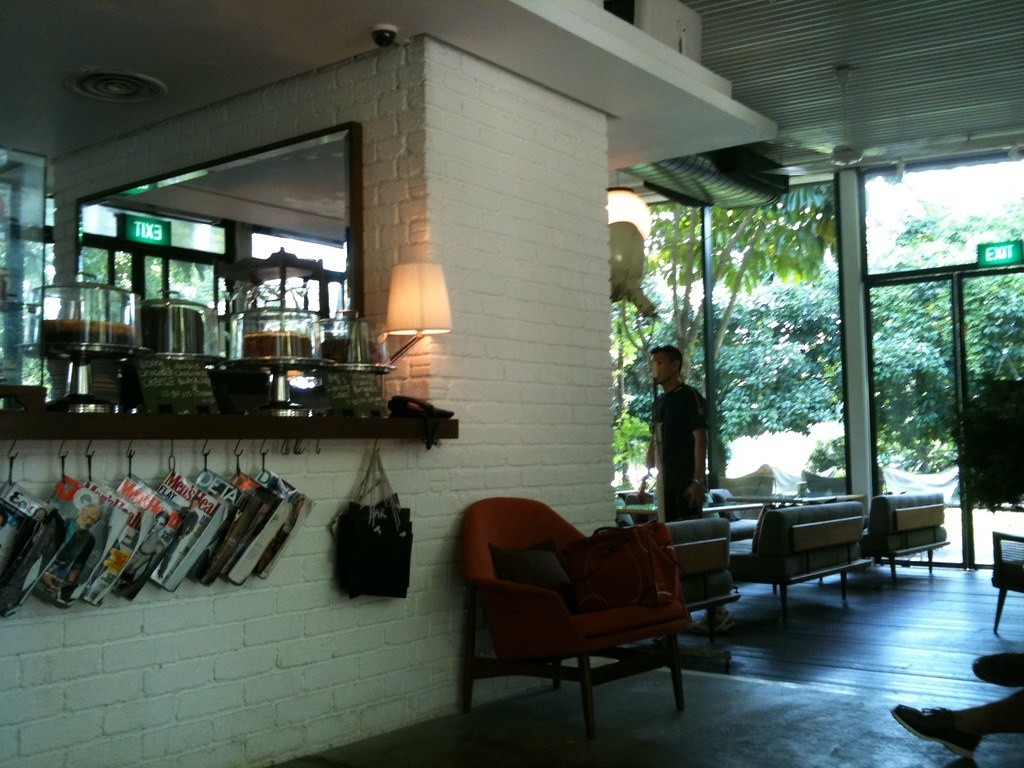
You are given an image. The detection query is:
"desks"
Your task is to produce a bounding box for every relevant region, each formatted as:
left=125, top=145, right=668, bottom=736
left=725, top=493, right=865, bottom=503
left=615, top=503, right=658, bottom=515
left=700, top=503, right=763, bottom=513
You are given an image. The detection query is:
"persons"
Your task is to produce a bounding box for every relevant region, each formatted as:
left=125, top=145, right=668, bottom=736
left=14, top=508, right=46, bottom=570
left=114, top=511, right=169, bottom=597
left=890, top=651, right=1024, bottom=755
left=643, top=345, right=737, bottom=630
left=39, top=502, right=99, bottom=601
left=157, top=497, right=198, bottom=582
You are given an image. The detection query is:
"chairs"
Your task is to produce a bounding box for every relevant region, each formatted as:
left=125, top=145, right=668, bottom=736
left=456, top=495, right=693, bottom=742
left=990, top=530, right=1024, bottom=634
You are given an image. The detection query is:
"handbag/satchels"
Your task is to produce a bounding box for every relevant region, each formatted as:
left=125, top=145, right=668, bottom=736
left=329, top=449, right=413, bottom=598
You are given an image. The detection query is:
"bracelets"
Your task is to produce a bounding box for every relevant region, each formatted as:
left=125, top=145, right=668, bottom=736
left=693, top=478, right=704, bottom=487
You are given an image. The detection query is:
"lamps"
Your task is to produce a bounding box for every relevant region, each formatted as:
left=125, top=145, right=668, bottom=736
left=605, top=168, right=652, bottom=241
left=385, top=261, right=452, bottom=364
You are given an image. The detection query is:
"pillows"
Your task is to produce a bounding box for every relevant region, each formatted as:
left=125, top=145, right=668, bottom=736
left=487, top=535, right=575, bottom=614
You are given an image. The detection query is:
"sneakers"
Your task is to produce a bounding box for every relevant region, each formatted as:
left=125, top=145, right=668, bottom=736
left=890, top=704, right=984, bottom=758
left=973, top=652, right=1024, bottom=686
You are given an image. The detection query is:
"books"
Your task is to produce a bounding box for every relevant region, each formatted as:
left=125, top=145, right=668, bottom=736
left=0, top=469, right=313, bottom=619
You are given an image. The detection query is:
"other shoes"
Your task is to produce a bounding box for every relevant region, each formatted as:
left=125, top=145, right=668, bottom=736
left=688, top=610, right=736, bottom=635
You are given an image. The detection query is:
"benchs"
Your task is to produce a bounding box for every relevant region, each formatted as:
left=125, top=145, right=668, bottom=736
left=615, top=489, right=951, bottom=646
